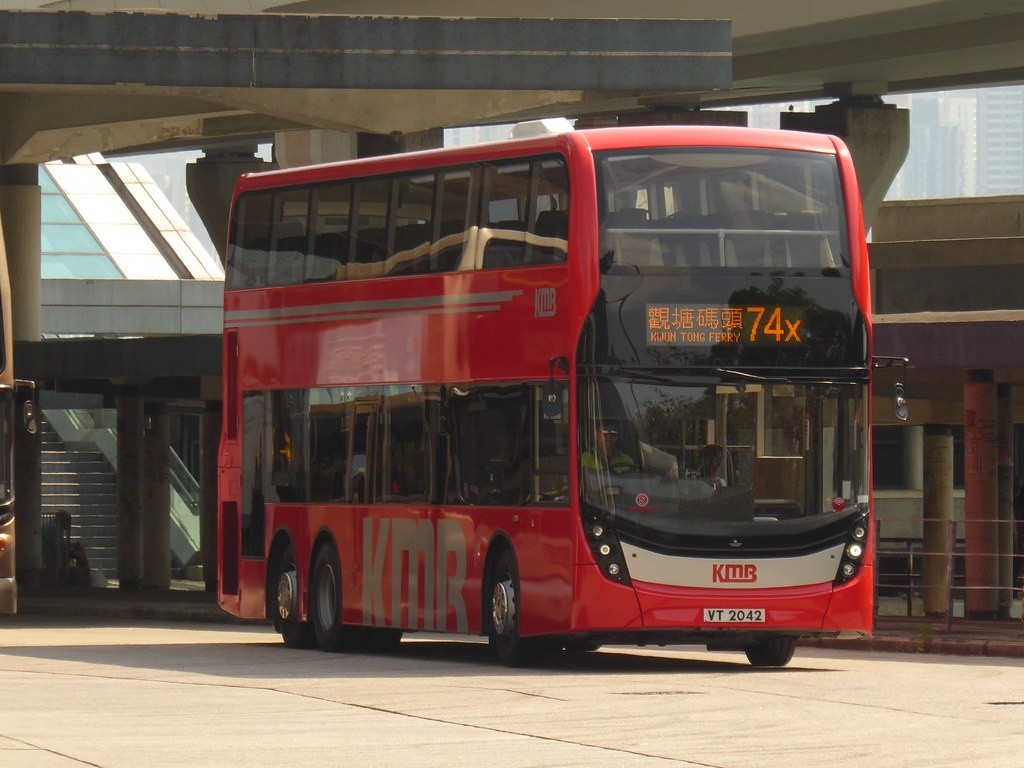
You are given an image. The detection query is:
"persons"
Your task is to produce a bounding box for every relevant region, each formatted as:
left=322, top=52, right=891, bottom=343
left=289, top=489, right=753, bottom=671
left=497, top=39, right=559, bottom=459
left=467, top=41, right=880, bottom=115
left=694, top=445, right=733, bottom=491
left=566, top=422, right=639, bottom=509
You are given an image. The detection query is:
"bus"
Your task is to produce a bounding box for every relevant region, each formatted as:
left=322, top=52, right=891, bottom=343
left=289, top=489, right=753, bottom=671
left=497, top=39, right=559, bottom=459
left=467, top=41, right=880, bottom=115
left=216, top=125, right=910, bottom=668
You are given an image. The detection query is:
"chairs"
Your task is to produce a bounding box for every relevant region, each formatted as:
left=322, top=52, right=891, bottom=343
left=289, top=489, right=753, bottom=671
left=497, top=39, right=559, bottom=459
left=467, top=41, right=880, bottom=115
left=235, top=207, right=821, bottom=289
